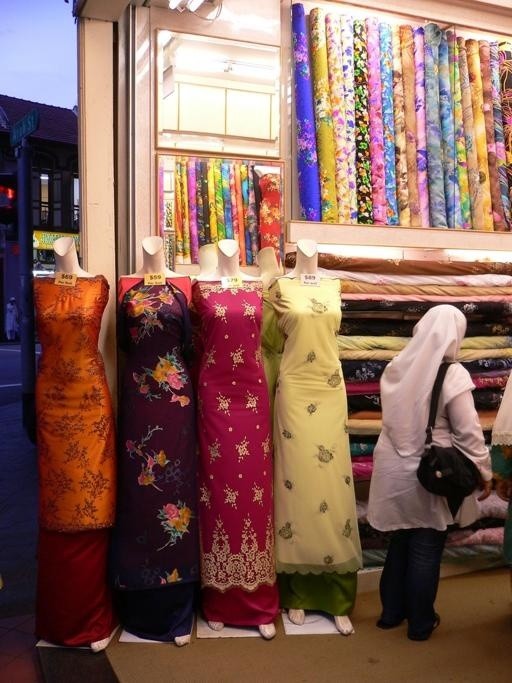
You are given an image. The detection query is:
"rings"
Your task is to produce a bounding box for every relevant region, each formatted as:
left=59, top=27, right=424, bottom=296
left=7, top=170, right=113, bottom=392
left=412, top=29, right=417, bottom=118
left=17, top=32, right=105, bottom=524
left=486, top=486, right=491, bottom=489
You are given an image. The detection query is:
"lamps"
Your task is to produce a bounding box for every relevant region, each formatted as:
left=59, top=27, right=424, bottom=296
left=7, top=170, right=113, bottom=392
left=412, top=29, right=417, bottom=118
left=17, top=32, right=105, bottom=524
left=166, top=0, right=205, bottom=15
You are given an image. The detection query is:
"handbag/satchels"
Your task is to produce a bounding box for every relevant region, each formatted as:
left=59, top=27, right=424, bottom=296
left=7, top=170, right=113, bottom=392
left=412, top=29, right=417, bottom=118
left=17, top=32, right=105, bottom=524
left=415, top=446, right=480, bottom=499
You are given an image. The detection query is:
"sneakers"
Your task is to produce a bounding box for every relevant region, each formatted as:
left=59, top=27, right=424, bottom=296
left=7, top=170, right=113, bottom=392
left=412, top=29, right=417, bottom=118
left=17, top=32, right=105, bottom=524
left=406, top=610, right=441, bottom=642
left=376, top=610, right=405, bottom=630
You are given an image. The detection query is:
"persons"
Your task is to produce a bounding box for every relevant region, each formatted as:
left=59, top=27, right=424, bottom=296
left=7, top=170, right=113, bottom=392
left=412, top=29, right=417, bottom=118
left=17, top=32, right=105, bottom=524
left=491, top=369, right=512, bottom=566
left=4, top=296, right=17, bottom=340
left=252, top=165, right=281, bottom=268
left=269, top=239, right=364, bottom=633
left=189, top=238, right=280, bottom=640
left=115, top=235, right=196, bottom=646
left=367, top=303, right=494, bottom=639
left=30, top=236, right=116, bottom=653
